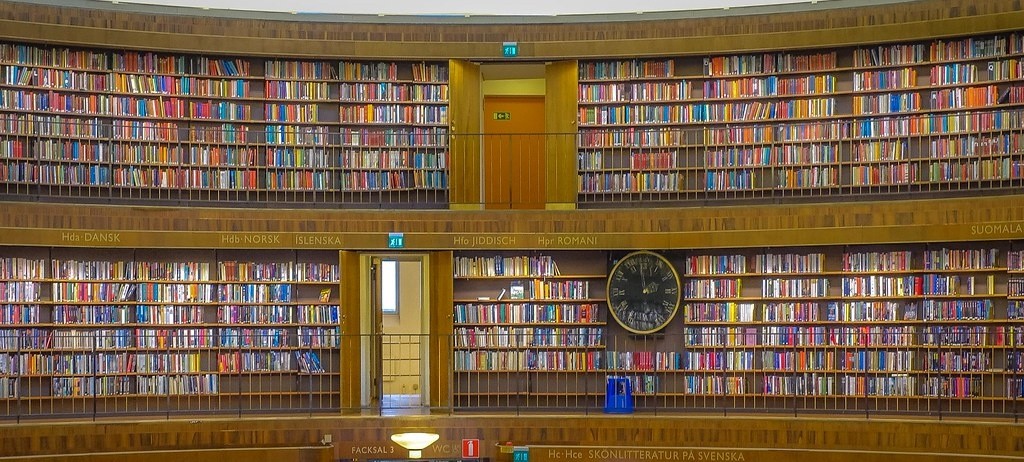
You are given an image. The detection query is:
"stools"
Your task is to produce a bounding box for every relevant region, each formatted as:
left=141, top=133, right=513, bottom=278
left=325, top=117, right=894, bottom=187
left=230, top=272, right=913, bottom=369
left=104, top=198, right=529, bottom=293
left=604, top=377, right=634, bottom=414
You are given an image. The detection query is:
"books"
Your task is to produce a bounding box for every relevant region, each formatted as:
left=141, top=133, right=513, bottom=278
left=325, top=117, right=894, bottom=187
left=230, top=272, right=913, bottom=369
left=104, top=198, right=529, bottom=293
left=218, top=251, right=342, bottom=375
left=1, top=41, right=258, bottom=190
left=607, top=352, right=683, bottom=394
left=0, top=252, right=217, bottom=397
left=454, top=254, right=604, bottom=373
left=266, top=60, right=449, bottom=192
left=695, top=52, right=850, bottom=189
left=578, top=59, right=693, bottom=194
left=853, top=35, right=1024, bottom=185
left=684, top=253, right=1024, bottom=397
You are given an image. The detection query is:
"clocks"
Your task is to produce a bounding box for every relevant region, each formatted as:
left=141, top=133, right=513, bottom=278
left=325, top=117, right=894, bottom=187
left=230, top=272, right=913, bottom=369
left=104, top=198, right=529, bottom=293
left=606, top=251, right=682, bottom=335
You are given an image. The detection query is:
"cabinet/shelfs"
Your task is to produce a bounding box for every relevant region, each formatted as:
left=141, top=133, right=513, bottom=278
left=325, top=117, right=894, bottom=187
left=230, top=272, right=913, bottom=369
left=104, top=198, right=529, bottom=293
left=0, top=29, right=1024, bottom=419
